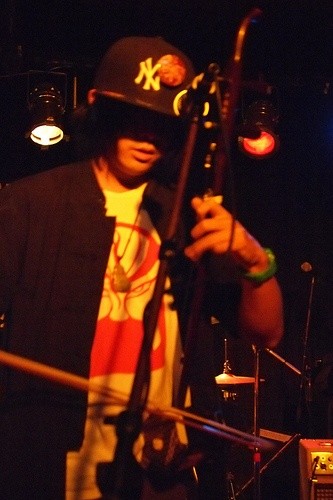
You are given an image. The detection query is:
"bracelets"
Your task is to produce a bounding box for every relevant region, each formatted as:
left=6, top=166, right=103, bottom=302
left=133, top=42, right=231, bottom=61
left=238, top=249, right=278, bottom=283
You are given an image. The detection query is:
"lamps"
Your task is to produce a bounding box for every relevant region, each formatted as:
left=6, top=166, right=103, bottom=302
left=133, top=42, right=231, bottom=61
left=23, top=69, right=71, bottom=153
left=237, top=99, right=283, bottom=161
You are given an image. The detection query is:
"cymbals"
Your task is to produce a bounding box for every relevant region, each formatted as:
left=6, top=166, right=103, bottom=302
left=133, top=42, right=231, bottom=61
left=214, top=373, right=266, bottom=385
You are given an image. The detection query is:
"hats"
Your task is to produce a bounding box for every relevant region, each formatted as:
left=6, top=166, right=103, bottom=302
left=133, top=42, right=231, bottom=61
left=89, top=36, right=196, bottom=123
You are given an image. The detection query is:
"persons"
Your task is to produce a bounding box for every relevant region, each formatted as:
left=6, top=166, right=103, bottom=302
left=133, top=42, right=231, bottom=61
left=0, top=35, right=284, bottom=500
